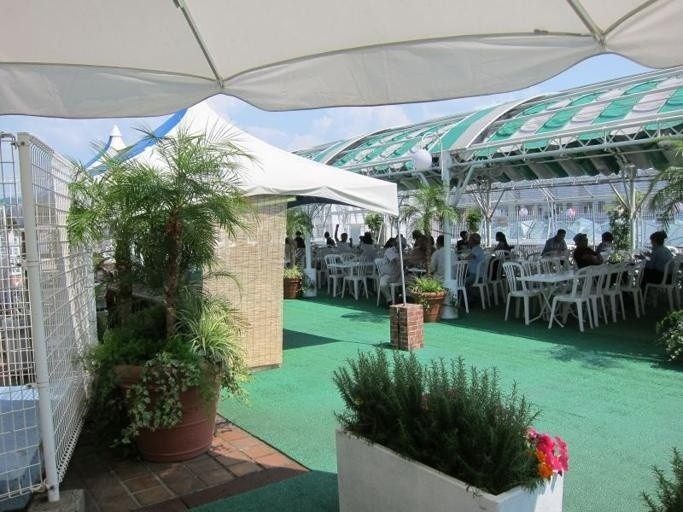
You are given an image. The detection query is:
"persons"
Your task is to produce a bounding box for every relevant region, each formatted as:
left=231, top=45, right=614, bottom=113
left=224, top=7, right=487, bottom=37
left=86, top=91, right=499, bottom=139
left=540, top=228, right=567, bottom=256
left=457, top=231, right=472, bottom=261
left=460, top=232, right=485, bottom=312
left=286, top=223, right=437, bottom=305
left=595, top=231, right=613, bottom=254
left=494, top=231, right=509, bottom=255
left=573, top=232, right=602, bottom=269
left=641, top=230, right=673, bottom=296
left=430, top=235, right=457, bottom=281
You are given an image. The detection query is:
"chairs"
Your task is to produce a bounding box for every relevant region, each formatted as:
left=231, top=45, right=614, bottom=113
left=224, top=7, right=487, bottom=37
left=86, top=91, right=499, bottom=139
left=315, top=246, right=683, bottom=332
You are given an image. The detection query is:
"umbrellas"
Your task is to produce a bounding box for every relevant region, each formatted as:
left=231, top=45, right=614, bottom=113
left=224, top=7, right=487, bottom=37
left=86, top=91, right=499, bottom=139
left=0, top=1, right=683, bottom=121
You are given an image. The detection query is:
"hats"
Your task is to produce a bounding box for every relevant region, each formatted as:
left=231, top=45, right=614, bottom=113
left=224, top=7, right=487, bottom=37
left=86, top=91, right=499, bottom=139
left=470, top=233, right=480, bottom=241
left=396, top=237, right=406, bottom=248
left=573, top=234, right=587, bottom=245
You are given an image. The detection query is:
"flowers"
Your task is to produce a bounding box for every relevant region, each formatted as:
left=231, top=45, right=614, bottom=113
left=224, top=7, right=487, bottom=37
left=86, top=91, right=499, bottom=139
left=332, top=341, right=570, bottom=495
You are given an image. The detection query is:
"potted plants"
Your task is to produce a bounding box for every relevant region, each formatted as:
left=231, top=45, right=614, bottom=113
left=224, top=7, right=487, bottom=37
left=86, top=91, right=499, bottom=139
left=397, top=178, right=464, bottom=323
left=283, top=207, right=314, bottom=299
left=106, top=117, right=264, bottom=463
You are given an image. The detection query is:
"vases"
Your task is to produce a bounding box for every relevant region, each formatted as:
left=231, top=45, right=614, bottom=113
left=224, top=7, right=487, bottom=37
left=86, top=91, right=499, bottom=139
left=335, top=426, right=565, bottom=512
